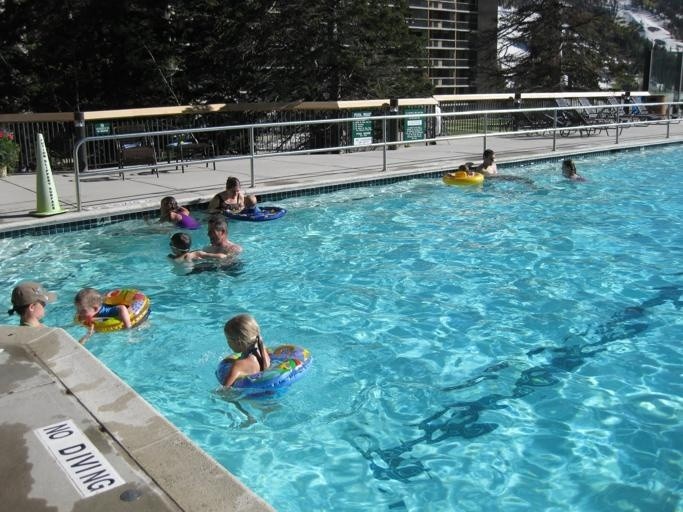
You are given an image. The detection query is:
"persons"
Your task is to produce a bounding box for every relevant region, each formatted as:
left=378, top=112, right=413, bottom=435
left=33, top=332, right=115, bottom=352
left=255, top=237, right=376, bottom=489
left=448, top=164, right=468, bottom=177
left=167, top=233, right=225, bottom=277
left=220, top=314, right=279, bottom=428
left=74, top=287, right=150, bottom=345
left=476, top=149, right=512, bottom=195
left=206, top=177, right=244, bottom=215
left=144, top=195, right=200, bottom=230
left=240, top=195, right=260, bottom=214
left=184, top=218, right=245, bottom=279
left=7, top=281, right=94, bottom=345
left=559, top=159, right=584, bottom=182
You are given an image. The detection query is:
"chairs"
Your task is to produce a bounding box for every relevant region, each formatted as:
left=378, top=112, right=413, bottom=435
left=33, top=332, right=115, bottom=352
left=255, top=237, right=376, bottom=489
left=175, top=115, right=216, bottom=172
left=521, top=95, right=680, bottom=138
left=112, top=124, right=160, bottom=180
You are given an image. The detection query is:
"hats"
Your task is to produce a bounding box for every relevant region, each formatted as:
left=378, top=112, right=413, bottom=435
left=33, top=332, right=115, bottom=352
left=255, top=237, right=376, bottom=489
left=12, top=281, right=57, bottom=309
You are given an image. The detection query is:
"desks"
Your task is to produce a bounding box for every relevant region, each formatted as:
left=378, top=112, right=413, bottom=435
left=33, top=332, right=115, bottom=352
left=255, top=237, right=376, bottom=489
left=165, top=141, right=192, bottom=164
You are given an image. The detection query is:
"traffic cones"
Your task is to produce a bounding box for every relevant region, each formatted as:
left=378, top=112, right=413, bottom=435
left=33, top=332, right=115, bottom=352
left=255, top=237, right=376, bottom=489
left=30, top=132, right=68, bottom=218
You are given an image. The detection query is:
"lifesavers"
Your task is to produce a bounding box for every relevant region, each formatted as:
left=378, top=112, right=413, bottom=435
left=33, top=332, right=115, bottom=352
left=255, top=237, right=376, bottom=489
left=442, top=169, right=484, bottom=185
left=215, top=344, right=314, bottom=391
left=76, top=287, right=150, bottom=332
left=224, top=205, right=286, bottom=221
